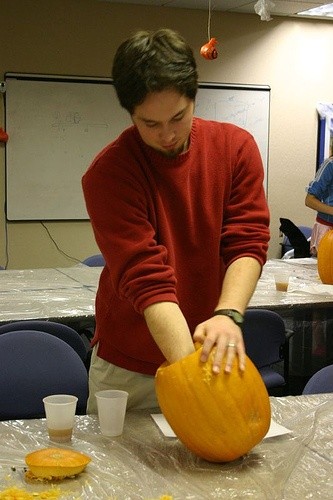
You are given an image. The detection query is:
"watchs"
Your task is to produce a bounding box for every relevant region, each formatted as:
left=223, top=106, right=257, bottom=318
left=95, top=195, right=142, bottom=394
left=214, top=309, right=244, bottom=327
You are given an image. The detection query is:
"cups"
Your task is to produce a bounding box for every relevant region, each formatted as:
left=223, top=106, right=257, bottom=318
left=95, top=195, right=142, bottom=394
left=94, top=390, right=129, bottom=437
left=43, top=394, right=78, bottom=444
left=273, top=271, right=290, bottom=292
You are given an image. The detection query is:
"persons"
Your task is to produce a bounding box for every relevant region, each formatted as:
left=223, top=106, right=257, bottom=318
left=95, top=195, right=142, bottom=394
left=81, top=28, right=270, bottom=415
left=305, top=137, right=333, bottom=258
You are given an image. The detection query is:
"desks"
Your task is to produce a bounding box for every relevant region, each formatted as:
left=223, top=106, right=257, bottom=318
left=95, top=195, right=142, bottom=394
left=0, top=255, right=333, bottom=396
left=0, top=392, right=333, bottom=500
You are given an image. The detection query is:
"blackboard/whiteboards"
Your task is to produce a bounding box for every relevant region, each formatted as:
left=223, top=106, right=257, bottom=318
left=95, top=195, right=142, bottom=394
left=4, top=72, right=269, bottom=222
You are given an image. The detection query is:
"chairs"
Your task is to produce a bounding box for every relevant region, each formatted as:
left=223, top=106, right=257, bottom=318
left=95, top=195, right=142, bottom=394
left=0, top=225, right=333, bottom=421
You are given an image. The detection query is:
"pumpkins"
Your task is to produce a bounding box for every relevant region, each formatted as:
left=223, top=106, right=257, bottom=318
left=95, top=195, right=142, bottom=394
left=317, top=229, right=333, bottom=285
left=155, top=342, right=271, bottom=462
left=25, top=447, right=90, bottom=480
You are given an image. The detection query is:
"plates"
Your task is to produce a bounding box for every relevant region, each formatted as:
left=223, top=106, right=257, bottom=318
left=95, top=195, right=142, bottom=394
left=24, top=447, right=91, bottom=480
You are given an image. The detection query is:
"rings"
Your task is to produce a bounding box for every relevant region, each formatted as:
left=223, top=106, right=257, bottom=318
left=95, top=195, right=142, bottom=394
left=228, top=343, right=237, bottom=348
